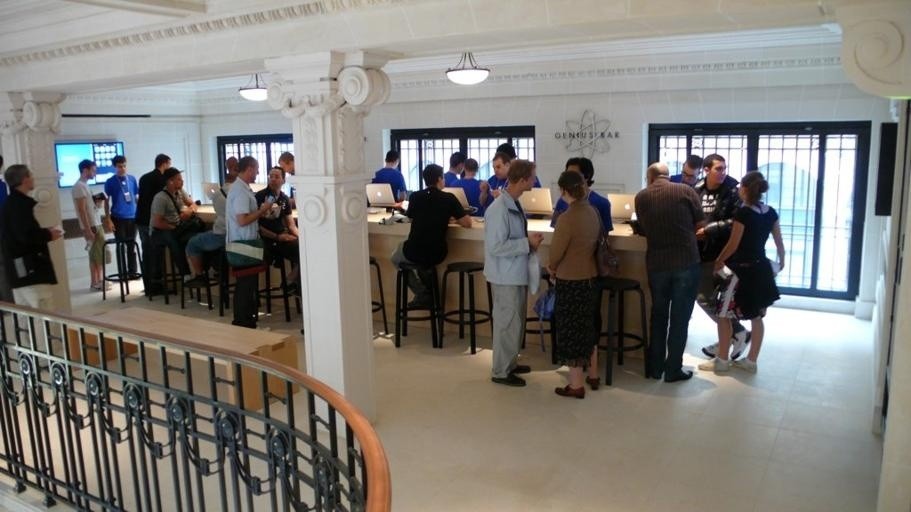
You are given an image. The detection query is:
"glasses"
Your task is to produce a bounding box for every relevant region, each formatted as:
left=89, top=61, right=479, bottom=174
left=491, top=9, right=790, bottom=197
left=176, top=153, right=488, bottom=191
left=681, top=172, right=700, bottom=179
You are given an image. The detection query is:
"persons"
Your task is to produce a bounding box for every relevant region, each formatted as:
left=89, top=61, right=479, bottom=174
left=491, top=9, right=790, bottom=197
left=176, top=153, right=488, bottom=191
left=696, top=154, right=751, bottom=360
left=483, top=159, right=544, bottom=386
left=135, top=154, right=172, bottom=297
left=390, top=164, right=472, bottom=307
left=443, top=144, right=544, bottom=220
left=0, top=164, right=62, bottom=289
left=549, top=170, right=604, bottom=398
left=375, top=150, right=407, bottom=202
left=71, top=159, right=112, bottom=290
left=104, top=156, right=140, bottom=279
left=635, top=162, right=704, bottom=384
left=226, top=156, right=271, bottom=329
left=254, top=166, right=300, bottom=293
left=550, top=157, right=613, bottom=276
left=670, top=156, right=704, bottom=186
left=185, top=167, right=238, bottom=286
left=698, top=171, right=785, bottom=375
left=278, top=152, right=297, bottom=208
left=149, top=166, right=198, bottom=274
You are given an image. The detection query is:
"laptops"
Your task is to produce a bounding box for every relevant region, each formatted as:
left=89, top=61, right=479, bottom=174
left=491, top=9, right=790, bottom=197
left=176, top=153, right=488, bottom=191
left=518, top=188, right=554, bottom=215
left=607, top=192, right=642, bottom=224
left=441, top=187, right=472, bottom=213
left=250, top=184, right=267, bottom=194
left=366, top=183, right=400, bottom=207
left=203, top=182, right=222, bottom=201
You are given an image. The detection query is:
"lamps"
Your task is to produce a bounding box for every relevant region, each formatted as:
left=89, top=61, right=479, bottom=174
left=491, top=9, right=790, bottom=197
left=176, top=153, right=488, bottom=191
left=445, top=51, right=490, bottom=85
left=238, top=73, right=268, bottom=102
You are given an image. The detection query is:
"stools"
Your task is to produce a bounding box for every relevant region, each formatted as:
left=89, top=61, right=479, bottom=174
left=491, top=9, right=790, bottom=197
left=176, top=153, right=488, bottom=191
left=521, top=266, right=556, bottom=365
left=102, top=237, right=389, bottom=335
left=597, top=276, right=651, bottom=386
left=394, top=262, right=441, bottom=349
left=439, top=262, right=494, bottom=355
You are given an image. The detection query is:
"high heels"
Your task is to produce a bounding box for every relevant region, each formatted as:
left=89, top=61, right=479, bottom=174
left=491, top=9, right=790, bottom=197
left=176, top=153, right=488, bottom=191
left=585, top=376, right=600, bottom=390
left=555, top=384, right=585, bottom=399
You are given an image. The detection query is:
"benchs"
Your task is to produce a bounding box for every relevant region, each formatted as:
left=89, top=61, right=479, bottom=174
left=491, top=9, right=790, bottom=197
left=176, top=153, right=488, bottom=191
left=65, top=306, right=300, bottom=415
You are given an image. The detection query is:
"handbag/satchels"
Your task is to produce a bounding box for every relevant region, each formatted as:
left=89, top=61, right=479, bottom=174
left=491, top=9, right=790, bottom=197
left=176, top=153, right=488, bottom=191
left=533, top=286, right=556, bottom=320
left=596, top=237, right=620, bottom=276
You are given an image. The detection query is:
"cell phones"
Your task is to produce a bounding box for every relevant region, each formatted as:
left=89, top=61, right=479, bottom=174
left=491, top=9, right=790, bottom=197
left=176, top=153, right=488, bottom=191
left=267, top=196, right=276, bottom=207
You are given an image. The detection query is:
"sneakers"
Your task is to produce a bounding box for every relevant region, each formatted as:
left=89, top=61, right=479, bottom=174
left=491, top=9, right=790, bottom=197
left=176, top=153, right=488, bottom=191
left=699, top=329, right=757, bottom=374
left=183, top=274, right=210, bottom=287
left=511, top=365, right=531, bottom=373
left=492, top=372, right=526, bottom=386
left=90, top=280, right=113, bottom=291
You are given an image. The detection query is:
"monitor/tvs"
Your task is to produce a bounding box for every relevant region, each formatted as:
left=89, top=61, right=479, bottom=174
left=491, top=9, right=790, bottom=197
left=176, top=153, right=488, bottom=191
left=55, top=141, right=126, bottom=188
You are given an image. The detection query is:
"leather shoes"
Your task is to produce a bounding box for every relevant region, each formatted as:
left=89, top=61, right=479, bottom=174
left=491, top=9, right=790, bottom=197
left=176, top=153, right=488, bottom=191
left=664, top=371, right=692, bottom=383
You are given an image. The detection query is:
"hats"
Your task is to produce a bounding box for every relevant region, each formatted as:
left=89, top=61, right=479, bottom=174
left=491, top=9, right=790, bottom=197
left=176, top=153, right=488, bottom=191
left=162, top=167, right=184, bottom=182
left=496, top=143, right=518, bottom=159
